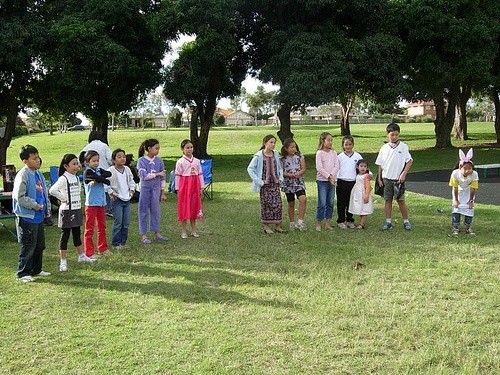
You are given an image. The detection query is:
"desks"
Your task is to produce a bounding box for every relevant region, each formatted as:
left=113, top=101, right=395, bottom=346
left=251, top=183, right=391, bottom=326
left=0, top=190, right=18, bottom=213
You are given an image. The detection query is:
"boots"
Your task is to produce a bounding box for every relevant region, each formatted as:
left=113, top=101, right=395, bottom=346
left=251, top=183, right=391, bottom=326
left=315, top=220, right=321, bottom=232
left=325, top=221, right=334, bottom=230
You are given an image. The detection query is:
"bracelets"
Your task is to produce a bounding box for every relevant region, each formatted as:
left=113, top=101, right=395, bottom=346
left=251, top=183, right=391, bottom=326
left=469, top=200, right=473, bottom=203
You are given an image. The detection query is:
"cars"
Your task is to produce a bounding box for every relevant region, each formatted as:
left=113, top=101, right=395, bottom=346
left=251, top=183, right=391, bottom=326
left=66, top=125, right=85, bottom=131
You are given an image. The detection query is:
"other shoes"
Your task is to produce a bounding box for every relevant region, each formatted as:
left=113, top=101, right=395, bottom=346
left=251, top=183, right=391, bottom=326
left=452, top=226, right=459, bottom=238
left=190, top=231, right=200, bottom=238
left=356, top=224, right=365, bottom=229
left=274, top=227, right=289, bottom=234
left=112, top=244, right=131, bottom=250
left=44, top=218, right=54, bottom=226
left=289, top=223, right=299, bottom=231
left=181, top=232, right=189, bottom=238
left=98, top=250, right=114, bottom=256
left=141, top=237, right=152, bottom=243
left=297, top=221, right=308, bottom=231
left=36, top=270, right=51, bottom=276
left=345, top=222, right=356, bottom=228
left=104, top=209, right=113, bottom=217
left=18, top=275, right=37, bottom=282
left=337, top=223, right=347, bottom=229
left=263, top=229, right=276, bottom=235
left=465, top=227, right=475, bottom=235
left=154, top=235, right=169, bottom=240
left=89, top=253, right=102, bottom=259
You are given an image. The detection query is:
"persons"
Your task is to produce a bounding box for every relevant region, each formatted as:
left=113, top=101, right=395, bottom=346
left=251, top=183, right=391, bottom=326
left=449, top=148, right=478, bottom=234
left=12, top=145, right=51, bottom=282
left=175, top=139, right=205, bottom=238
left=316, top=132, right=340, bottom=231
left=137, top=139, right=168, bottom=243
left=49, top=154, right=91, bottom=271
left=125, top=155, right=140, bottom=201
left=247, top=135, right=284, bottom=234
left=104, top=149, right=136, bottom=248
left=79, top=150, right=112, bottom=258
left=336, top=136, right=373, bottom=228
left=375, top=123, right=413, bottom=229
left=78, top=130, right=113, bottom=216
left=348, top=160, right=373, bottom=229
left=280, top=138, right=308, bottom=230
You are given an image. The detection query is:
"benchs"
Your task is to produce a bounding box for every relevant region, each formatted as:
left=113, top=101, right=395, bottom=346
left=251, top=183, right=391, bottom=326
left=0, top=213, right=18, bottom=239
left=474, top=164, right=500, bottom=178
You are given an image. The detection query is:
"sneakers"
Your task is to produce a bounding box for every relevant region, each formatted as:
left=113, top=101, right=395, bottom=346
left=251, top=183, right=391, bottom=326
left=404, top=221, right=412, bottom=230
left=78, top=254, right=94, bottom=263
left=59, top=264, right=68, bottom=272
left=382, top=221, right=393, bottom=230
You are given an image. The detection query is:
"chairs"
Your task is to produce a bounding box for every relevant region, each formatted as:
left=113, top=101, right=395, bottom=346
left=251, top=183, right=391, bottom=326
left=197, top=159, right=213, bottom=202
left=45, top=166, right=62, bottom=215
